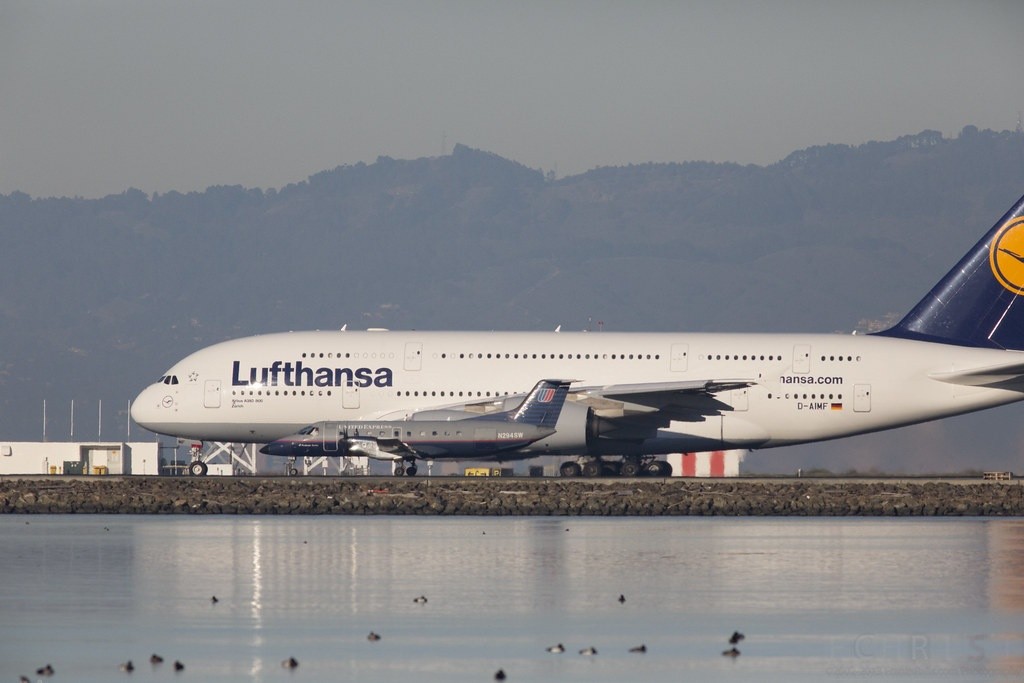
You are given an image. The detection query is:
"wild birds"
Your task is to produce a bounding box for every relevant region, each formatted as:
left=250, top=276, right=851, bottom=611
left=366, top=631, right=382, bottom=641
left=413, top=595, right=428, bottom=606
left=123, top=655, right=185, bottom=674
left=210, top=595, right=220, bottom=604
left=628, top=644, right=647, bottom=653
left=35, top=663, right=54, bottom=677
left=721, top=630, right=746, bottom=658
left=546, top=643, right=566, bottom=654
left=580, top=646, right=597, bottom=657
left=283, top=656, right=299, bottom=669
left=617, top=593, right=628, bottom=604
left=495, top=669, right=506, bottom=681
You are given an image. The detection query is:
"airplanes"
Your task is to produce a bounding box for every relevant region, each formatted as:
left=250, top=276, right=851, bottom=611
left=129, top=196, right=1024, bottom=477
